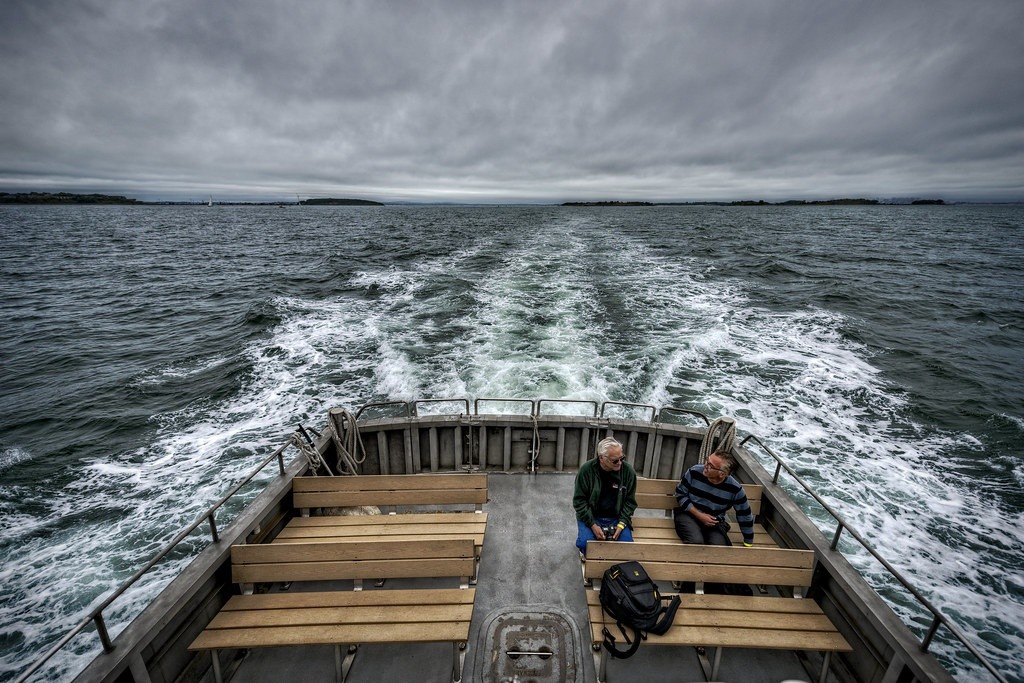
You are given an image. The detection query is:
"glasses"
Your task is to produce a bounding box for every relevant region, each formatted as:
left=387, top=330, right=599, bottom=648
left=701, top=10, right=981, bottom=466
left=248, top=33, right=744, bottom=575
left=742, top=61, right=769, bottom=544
left=704, top=458, right=727, bottom=474
left=602, top=453, right=628, bottom=465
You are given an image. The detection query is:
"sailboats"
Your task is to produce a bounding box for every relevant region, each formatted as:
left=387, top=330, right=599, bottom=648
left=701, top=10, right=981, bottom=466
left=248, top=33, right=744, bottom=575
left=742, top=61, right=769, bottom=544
left=208, top=195, right=213, bottom=207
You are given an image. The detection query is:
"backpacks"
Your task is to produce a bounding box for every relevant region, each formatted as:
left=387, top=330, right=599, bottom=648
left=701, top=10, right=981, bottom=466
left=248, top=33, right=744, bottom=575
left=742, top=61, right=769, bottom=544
left=598, top=561, right=682, bottom=658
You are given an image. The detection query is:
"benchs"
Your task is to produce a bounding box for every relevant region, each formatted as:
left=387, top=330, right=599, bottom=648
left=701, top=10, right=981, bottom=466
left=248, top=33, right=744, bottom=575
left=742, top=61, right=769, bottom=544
left=269, top=473, right=488, bottom=590
left=187, top=539, right=476, bottom=683
left=586, top=540, right=853, bottom=683
left=579, top=479, right=781, bottom=594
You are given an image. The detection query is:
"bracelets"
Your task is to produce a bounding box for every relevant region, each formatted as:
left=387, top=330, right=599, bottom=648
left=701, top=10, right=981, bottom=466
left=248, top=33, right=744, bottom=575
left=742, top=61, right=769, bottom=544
left=617, top=523, right=624, bottom=529
left=744, top=543, right=753, bottom=546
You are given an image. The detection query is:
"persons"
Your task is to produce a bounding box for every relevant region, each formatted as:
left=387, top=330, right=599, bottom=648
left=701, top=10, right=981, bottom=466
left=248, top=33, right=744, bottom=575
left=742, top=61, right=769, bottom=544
left=674, top=450, right=754, bottom=594
left=572, top=437, right=637, bottom=550
